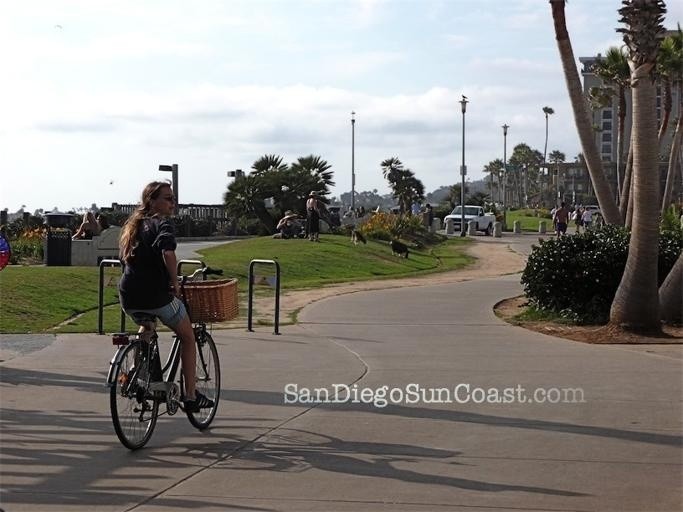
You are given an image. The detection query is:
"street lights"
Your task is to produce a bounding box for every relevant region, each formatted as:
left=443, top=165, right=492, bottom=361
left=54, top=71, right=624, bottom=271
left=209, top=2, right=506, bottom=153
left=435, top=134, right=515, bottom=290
left=351, top=111, right=356, bottom=209
left=458, top=98, right=469, bottom=236
left=500, top=124, right=510, bottom=222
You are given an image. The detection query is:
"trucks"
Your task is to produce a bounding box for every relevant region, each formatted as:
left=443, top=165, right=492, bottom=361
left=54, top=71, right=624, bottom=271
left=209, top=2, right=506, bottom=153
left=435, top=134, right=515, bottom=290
left=327, top=204, right=349, bottom=221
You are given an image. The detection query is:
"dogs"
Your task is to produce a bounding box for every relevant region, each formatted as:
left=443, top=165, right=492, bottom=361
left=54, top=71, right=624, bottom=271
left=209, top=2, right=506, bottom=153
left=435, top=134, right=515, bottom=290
left=390, top=239, right=409, bottom=258
left=350, top=229, right=366, bottom=245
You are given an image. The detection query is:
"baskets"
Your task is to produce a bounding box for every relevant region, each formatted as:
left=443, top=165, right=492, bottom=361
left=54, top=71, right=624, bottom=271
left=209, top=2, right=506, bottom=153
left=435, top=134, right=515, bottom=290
left=175, top=278, right=239, bottom=322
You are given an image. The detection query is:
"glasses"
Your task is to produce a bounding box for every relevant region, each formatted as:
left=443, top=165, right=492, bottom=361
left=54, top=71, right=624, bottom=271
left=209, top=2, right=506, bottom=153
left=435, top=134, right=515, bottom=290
left=160, top=195, right=177, bottom=201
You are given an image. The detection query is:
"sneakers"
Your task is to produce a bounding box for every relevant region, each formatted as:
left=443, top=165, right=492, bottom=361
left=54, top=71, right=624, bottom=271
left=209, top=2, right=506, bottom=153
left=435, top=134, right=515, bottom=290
left=183, top=389, right=215, bottom=408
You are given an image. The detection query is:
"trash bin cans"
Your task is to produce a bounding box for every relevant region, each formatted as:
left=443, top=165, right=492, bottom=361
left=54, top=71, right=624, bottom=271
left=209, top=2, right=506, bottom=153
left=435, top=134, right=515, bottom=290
left=47, top=231, right=72, bottom=266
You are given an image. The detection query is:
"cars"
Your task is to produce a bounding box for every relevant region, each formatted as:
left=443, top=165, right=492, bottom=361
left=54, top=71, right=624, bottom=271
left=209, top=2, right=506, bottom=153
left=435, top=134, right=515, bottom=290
left=390, top=204, right=401, bottom=214
left=583, top=205, right=601, bottom=215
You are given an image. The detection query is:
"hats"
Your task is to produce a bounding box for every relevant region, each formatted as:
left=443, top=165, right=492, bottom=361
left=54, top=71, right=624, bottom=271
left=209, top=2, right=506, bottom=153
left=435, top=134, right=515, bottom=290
left=309, top=190, right=317, bottom=196
left=282, top=210, right=298, bottom=219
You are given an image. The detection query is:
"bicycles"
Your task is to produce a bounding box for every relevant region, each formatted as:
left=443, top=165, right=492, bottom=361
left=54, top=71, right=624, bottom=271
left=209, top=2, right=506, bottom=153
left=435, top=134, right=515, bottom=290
left=104, top=267, right=239, bottom=450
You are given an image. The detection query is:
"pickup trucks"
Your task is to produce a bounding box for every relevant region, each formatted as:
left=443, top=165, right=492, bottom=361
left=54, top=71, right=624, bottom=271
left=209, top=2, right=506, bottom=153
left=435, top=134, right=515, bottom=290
left=444, top=205, right=496, bottom=235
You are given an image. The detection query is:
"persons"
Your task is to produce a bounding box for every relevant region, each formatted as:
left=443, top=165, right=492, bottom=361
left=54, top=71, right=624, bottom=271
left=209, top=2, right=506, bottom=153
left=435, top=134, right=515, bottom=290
left=118, top=181, right=216, bottom=413
left=550, top=203, right=592, bottom=237
left=71, top=212, right=110, bottom=241
left=343, top=204, right=370, bottom=224
left=272, top=190, right=321, bottom=242
left=410, top=200, right=433, bottom=226
